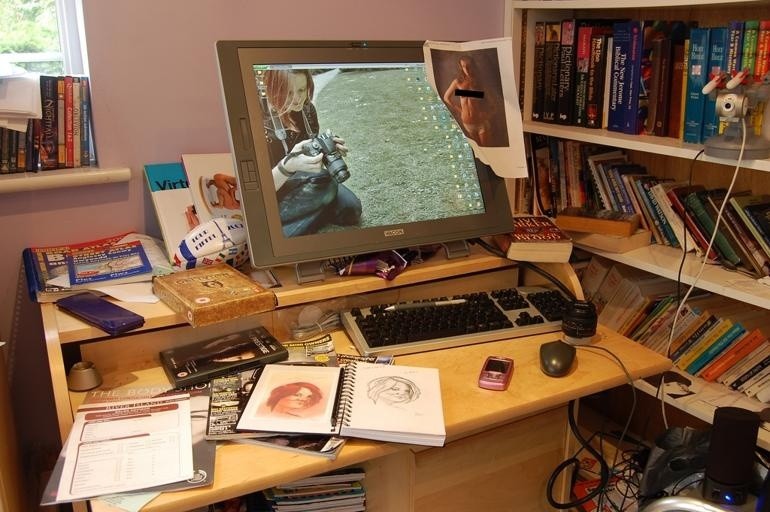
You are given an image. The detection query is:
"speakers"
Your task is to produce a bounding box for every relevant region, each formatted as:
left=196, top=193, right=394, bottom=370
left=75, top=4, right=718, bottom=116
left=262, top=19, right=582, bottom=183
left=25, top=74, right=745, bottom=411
left=703, top=407, right=760, bottom=505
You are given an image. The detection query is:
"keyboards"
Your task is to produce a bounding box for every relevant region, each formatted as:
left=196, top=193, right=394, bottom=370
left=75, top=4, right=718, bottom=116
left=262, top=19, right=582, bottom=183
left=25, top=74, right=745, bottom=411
left=340, top=287, right=571, bottom=358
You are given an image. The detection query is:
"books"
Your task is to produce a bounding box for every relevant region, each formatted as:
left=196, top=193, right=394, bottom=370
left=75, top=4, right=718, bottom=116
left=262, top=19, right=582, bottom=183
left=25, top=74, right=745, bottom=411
left=70, top=239, right=154, bottom=287
left=22, top=234, right=148, bottom=303
left=572, top=253, right=769, bottom=406
left=516, top=140, right=769, bottom=278
left=494, top=216, right=573, bottom=264
left=532, top=16, right=769, bottom=145
left=40, top=326, right=445, bottom=512
left=0, top=63, right=90, bottom=174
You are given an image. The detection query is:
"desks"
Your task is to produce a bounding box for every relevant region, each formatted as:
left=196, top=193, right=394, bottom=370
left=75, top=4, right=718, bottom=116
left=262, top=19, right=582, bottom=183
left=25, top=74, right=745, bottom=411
left=37, top=231, right=674, bottom=512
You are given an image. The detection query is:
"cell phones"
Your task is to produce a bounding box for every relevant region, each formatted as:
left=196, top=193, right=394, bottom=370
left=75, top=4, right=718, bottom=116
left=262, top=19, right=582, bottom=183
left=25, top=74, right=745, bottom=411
left=478, top=356, right=514, bottom=391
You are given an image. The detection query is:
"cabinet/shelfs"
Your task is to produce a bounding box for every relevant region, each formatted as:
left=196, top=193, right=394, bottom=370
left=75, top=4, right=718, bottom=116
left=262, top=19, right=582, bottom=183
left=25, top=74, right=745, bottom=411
left=500, top=2, right=769, bottom=511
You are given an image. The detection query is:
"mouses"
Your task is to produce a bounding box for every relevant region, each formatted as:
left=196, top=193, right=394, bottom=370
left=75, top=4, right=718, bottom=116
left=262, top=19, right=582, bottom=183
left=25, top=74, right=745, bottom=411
left=540, top=340, right=576, bottom=376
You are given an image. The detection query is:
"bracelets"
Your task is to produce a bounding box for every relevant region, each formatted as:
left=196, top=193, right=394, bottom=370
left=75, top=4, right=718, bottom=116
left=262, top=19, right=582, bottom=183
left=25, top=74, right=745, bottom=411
left=278, top=160, right=296, bottom=177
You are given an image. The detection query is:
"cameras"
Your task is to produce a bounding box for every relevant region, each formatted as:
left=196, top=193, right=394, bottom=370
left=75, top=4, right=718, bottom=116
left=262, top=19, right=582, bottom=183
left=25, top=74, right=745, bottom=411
left=302, top=128, right=351, bottom=183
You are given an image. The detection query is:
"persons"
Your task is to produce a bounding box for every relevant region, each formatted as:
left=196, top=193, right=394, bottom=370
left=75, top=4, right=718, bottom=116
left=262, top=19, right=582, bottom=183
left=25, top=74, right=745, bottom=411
left=206, top=173, right=237, bottom=191
left=211, top=188, right=240, bottom=211
left=443, top=54, right=498, bottom=146
left=259, top=69, right=363, bottom=237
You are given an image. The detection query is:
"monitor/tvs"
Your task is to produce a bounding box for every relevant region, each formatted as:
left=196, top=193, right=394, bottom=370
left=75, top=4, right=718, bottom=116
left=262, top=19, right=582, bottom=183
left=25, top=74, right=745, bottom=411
left=216, top=40, right=515, bottom=285
